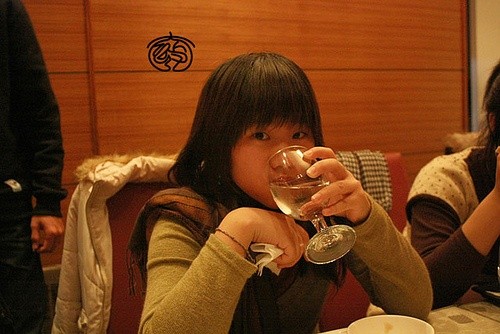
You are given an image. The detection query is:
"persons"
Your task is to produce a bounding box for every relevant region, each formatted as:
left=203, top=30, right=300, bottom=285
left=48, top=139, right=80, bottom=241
left=0, top=0, right=67, bottom=334
left=403, top=63, right=499, bottom=310
left=127, top=52, right=433, bottom=334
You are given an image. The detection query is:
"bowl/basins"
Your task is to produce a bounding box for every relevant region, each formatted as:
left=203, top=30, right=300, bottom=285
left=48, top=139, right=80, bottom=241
left=347, top=315, right=435, bottom=334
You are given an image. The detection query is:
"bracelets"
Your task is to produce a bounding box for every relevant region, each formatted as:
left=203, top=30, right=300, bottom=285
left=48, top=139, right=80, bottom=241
left=214, top=228, right=256, bottom=264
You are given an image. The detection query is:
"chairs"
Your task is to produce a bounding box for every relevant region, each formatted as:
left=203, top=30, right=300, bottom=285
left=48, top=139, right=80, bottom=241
left=313, top=149, right=407, bottom=332
left=51, top=153, right=186, bottom=334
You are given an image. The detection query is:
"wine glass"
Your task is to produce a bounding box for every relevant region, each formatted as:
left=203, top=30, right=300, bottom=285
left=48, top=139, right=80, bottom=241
left=268, top=145, right=357, bottom=265
